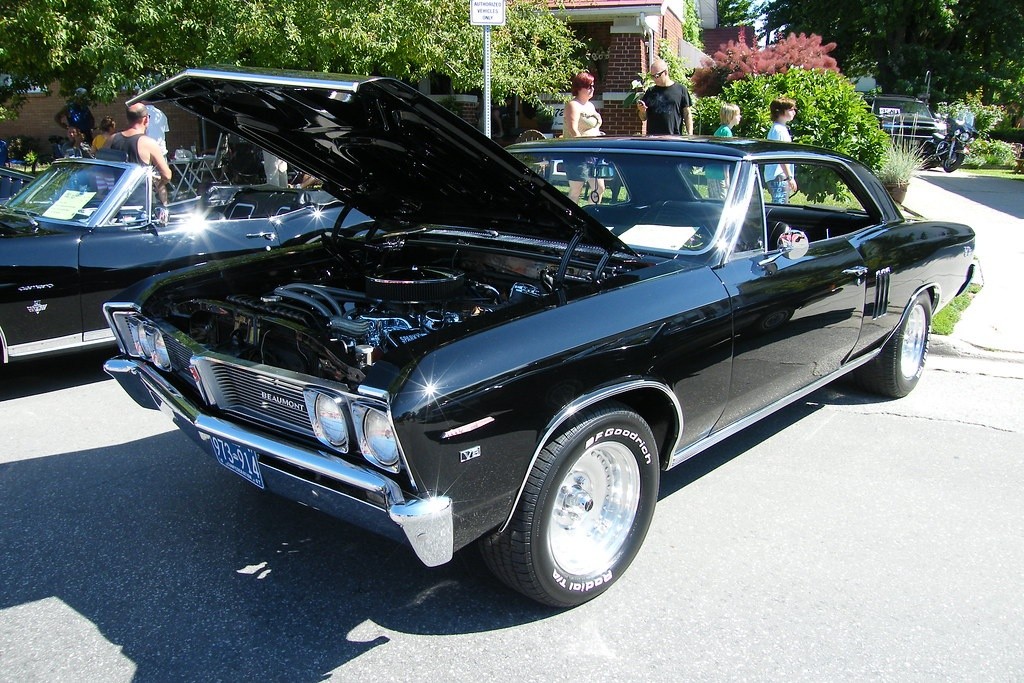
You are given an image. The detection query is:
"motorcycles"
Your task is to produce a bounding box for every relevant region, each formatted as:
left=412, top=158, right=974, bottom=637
left=920, top=106, right=979, bottom=173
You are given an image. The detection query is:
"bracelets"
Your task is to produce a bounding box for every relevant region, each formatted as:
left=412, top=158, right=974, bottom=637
left=784, top=176, right=792, bottom=181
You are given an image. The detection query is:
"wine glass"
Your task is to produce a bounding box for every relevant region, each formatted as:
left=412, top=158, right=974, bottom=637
left=191, top=146, right=196, bottom=159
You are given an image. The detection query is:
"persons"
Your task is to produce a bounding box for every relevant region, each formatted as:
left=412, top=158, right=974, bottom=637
left=563, top=73, right=605, bottom=204
left=637, top=60, right=693, bottom=135
left=262, top=150, right=288, bottom=187
left=295, top=173, right=317, bottom=188
left=218, top=133, right=260, bottom=184
left=101, top=103, right=172, bottom=206
left=138, top=88, right=170, bottom=206
left=91, top=116, right=115, bottom=189
left=55, top=88, right=95, bottom=158
left=764, top=97, right=798, bottom=204
left=704, top=103, right=742, bottom=199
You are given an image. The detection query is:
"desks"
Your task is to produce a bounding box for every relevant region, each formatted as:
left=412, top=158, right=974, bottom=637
left=166, top=157, right=218, bottom=203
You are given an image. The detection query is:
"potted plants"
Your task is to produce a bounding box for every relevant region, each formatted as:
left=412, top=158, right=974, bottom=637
left=872, top=110, right=928, bottom=205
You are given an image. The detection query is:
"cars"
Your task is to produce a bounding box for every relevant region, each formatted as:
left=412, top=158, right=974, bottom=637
left=857, top=92, right=948, bottom=153
left=102, top=68, right=977, bottom=609
left=0, top=157, right=385, bottom=390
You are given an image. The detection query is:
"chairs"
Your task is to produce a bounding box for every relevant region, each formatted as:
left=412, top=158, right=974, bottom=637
left=653, top=199, right=742, bottom=251
left=0, top=136, right=72, bottom=175
left=579, top=202, right=654, bottom=226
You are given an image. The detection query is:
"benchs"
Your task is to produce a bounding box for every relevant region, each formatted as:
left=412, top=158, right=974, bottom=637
left=223, top=189, right=315, bottom=224
left=763, top=204, right=868, bottom=251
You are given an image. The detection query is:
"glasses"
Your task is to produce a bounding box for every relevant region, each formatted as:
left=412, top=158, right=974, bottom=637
left=144, top=114, right=151, bottom=119
left=588, top=84, right=595, bottom=89
left=650, top=70, right=666, bottom=78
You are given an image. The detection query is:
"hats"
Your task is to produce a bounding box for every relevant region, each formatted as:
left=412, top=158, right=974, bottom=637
left=74, top=88, right=91, bottom=100
